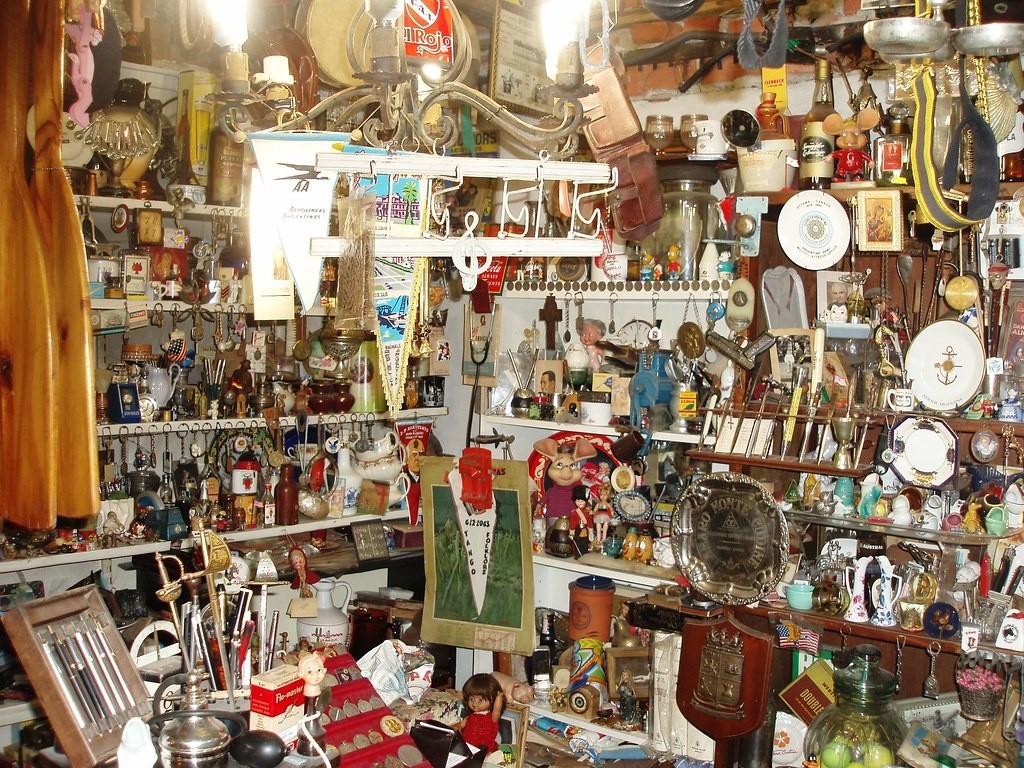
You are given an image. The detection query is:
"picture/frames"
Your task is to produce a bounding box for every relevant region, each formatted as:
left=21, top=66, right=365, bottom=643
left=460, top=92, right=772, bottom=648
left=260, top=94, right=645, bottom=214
left=858, top=190, right=905, bottom=252
left=457, top=697, right=530, bottom=768
left=605, top=648, right=652, bottom=697
left=767, top=329, right=815, bottom=394
left=894, top=692, right=977, bottom=740
left=817, top=270, right=864, bottom=321
left=486, top=0, right=555, bottom=119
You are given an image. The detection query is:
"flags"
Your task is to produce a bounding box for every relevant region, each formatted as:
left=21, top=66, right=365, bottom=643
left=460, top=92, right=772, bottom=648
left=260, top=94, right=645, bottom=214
left=776, top=623, right=819, bottom=654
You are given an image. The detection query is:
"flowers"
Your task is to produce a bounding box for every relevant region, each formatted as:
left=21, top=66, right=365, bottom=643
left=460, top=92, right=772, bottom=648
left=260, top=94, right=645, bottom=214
left=956, top=665, right=1005, bottom=690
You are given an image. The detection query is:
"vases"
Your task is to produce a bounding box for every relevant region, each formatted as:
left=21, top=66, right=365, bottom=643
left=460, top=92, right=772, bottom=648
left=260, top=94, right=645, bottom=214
left=334, top=447, right=365, bottom=516
left=958, top=685, right=1004, bottom=722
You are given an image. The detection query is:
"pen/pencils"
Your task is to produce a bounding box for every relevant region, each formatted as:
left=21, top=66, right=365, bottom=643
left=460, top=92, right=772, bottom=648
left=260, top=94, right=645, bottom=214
left=204, top=359, right=226, bottom=400
left=178, top=584, right=279, bottom=691
left=37, top=612, right=143, bottom=743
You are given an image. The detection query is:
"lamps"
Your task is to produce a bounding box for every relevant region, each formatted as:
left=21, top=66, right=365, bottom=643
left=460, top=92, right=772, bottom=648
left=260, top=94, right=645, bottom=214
left=209, top=0, right=600, bottom=160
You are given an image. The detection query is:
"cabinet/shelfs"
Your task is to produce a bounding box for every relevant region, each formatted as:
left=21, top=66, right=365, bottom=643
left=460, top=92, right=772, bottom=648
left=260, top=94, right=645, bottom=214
left=679, top=181, right=1024, bottom=714
left=0, top=0, right=450, bottom=726
left=433, top=281, right=733, bottom=744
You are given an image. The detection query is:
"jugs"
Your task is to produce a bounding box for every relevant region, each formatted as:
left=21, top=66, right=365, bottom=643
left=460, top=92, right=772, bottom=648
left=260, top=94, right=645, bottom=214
left=844, top=555, right=873, bottom=623
left=145, top=362, right=181, bottom=407
left=870, top=556, right=902, bottom=627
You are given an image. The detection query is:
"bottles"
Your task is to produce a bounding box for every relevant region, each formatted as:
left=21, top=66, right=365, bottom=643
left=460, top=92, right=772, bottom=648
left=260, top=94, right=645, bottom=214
left=164, top=263, right=180, bottom=300
left=209, top=104, right=243, bottom=205
left=296, top=577, right=352, bottom=646
left=804, top=642, right=909, bottom=768
left=874, top=115, right=914, bottom=186
left=163, top=456, right=221, bottom=529
left=634, top=164, right=717, bottom=282
left=738, top=96, right=797, bottom=194
left=154, top=667, right=229, bottom=768
left=259, top=483, right=275, bottom=528
left=274, top=460, right=299, bottom=525
left=331, top=429, right=362, bottom=516
left=798, top=58, right=839, bottom=190
left=540, top=610, right=557, bottom=666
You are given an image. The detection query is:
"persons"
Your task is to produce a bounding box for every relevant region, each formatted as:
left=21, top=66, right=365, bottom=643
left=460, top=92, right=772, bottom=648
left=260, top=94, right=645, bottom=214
left=640, top=246, right=681, bottom=282
left=452, top=673, right=507, bottom=757
left=794, top=342, right=804, bottom=365
left=617, top=670, right=635, bottom=719
left=289, top=544, right=321, bottom=598
left=569, top=462, right=615, bottom=561
left=827, top=283, right=848, bottom=312
left=296, top=655, right=327, bottom=697
left=103, top=511, right=125, bottom=536
left=514, top=258, right=534, bottom=283
left=580, top=319, right=607, bottom=385
left=213, top=514, right=234, bottom=532
left=540, top=371, right=556, bottom=395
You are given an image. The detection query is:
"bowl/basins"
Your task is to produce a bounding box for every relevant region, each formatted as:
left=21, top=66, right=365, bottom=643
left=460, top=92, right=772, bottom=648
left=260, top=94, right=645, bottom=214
left=785, top=585, right=814, bottom=610
left=166, top=185, right=207, bottom=203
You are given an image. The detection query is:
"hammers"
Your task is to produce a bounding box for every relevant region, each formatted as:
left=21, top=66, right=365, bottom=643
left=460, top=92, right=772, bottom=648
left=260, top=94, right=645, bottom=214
left=743, top=375, right=792, bottom=459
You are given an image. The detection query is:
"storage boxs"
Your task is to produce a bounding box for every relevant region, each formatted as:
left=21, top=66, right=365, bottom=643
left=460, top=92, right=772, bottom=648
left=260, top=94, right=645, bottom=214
left=384, top=519, right=423, bottom=548
left=487, top=223, right=543, bottom=280
left=357, top=479, right=389, bottom=516
left=249, top=664, right=305, bottom=751
left=778, top=657, right=836, bottom=725
left=592, top=373, right=619, bottom=392
left=147, top=508, right=188, bottom=541
left=139, top=657, right=183, bottom=726
left=178, top=71, right=220, bottom=187
left=679, top=391, right=700, bottom=417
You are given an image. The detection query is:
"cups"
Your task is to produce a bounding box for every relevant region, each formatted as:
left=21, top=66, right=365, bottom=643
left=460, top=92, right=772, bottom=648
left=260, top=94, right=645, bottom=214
left=419, top=376, right=445, bottom=407
left=354, top=430, right=410, bottom=505
left=287, top=443, right=324, bottom=463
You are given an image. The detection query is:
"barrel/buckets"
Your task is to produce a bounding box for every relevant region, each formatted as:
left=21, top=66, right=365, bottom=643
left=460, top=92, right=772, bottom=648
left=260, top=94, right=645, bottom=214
left=956, top=648, right=1008, bottom=722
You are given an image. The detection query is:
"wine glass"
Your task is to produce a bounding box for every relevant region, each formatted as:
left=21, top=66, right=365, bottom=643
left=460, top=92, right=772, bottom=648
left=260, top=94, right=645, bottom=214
left=681, top=114, right=707, bottom=154
left=645, top=115, right=675, bottom=155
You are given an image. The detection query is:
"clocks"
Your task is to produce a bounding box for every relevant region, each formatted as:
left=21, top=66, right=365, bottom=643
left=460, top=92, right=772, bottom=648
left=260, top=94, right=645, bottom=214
left=617, top=319, right=653, bottom=351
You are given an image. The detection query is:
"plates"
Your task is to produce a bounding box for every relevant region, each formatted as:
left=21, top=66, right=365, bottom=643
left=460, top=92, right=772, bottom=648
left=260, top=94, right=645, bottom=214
left=888, top=414, right=959, bottom=489
left=904, top=320, right=987, bottom=415
left=770, top=712, right=809, bottom=767
